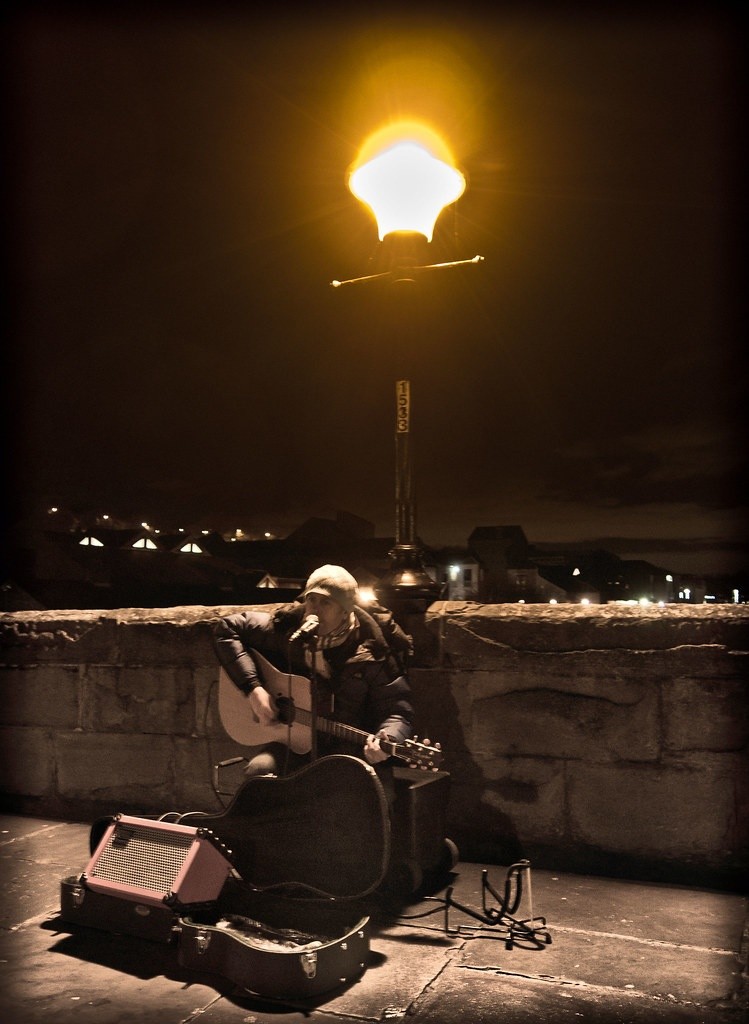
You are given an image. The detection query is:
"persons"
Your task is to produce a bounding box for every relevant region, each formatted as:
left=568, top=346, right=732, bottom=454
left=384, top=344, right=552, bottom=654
left=211, top=564, right=416, bottom=826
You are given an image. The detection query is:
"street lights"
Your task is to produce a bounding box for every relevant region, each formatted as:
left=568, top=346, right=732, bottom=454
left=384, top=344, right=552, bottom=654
left=328, top=116, right=488, bottom=765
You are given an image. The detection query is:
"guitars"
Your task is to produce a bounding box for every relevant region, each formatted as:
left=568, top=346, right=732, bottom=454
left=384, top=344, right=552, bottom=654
left=216, top=639, right=443, bottom=775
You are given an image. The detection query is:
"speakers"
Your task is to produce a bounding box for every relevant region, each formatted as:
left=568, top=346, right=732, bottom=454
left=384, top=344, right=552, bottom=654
left=74, top=813, right=234, bottom=943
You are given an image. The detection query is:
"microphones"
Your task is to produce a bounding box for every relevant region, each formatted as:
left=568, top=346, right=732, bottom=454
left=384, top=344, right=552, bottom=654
left=289, top=614, right=319, bottom=643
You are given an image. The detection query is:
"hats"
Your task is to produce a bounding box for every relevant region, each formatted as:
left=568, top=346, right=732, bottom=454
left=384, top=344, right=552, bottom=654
left=296, top=564, right=358, bottom=613
